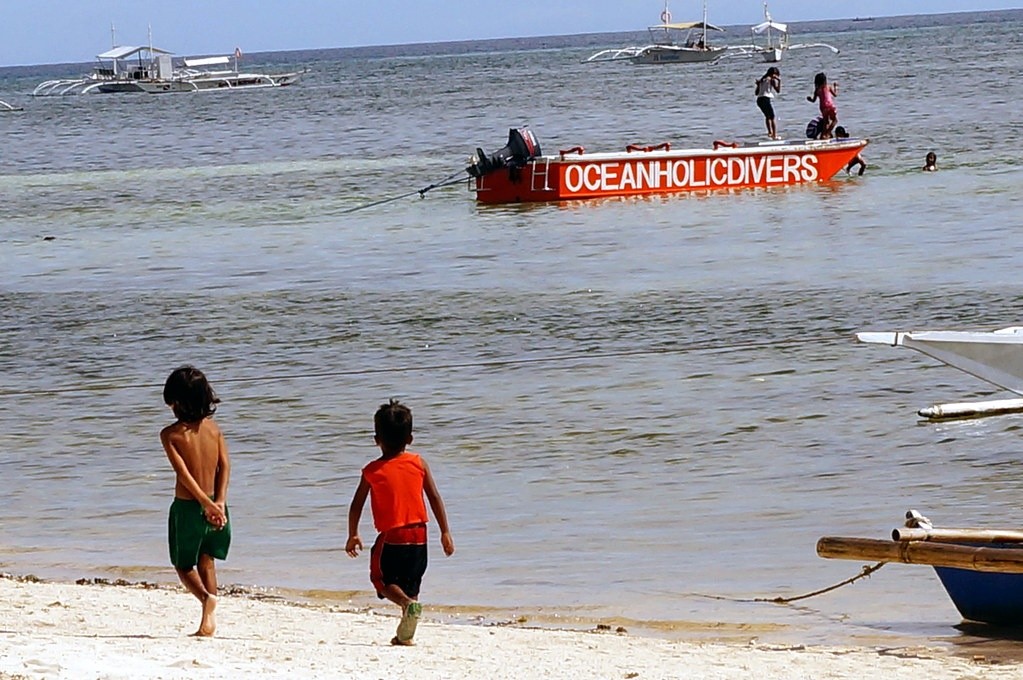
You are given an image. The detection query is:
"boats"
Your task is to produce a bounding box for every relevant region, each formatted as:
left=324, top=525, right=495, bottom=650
left=854, top=326, right=1023, bottom=399
left=751, top=3, right=789, bottom=62
left=467, top=124, right=869, bottom=205
left=818, top=509, right=1023, bottom=635
left=87, top=20, right=312, bottom=91
left=851, top=15, right=876, bottom=23
left=580, top=1, right=730, bottom=66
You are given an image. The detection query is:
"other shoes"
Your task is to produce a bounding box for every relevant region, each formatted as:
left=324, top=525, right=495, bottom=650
left=397, top=600, right=421, bottom=642
left=391, top=636, right=416, bottom=647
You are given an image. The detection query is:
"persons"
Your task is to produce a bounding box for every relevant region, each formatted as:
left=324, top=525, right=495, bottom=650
left=755, top=67, right=781, bottom=140
left=162, top=367, right=231, bottom=636
left=345, top=400, right=455, bottom=644
left=923, top=152, right=936, bottom=170
left=835, top=126, right=866, bottom=175
left=806, top=115, right=823, bottom=140
left=807, top=73, right=838, bottom=139
